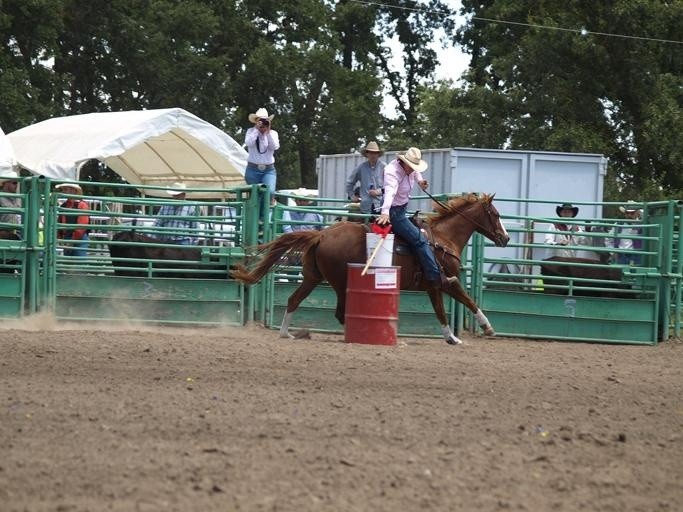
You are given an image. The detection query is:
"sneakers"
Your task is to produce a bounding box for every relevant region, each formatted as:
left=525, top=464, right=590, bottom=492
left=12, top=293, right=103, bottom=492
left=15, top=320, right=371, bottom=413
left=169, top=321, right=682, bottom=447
left=433, top=275, right=457, bottom=290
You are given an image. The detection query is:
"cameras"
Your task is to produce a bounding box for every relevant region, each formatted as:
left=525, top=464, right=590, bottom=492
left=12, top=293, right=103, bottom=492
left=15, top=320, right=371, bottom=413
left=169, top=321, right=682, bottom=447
left=260, top=117, right=269, bottom=128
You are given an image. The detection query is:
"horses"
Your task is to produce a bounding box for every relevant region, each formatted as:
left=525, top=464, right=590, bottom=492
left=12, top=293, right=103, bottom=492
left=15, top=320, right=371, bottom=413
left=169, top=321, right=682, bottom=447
left=224, top=191, right=511, bottom=347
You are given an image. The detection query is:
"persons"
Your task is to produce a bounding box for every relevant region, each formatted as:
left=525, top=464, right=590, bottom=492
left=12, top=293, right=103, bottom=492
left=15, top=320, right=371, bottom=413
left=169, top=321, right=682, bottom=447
left=606, top=198, right=643, bottom=269
left=375, top=147, right=457, bottom=290
left=346, top=142, right=388, bottom=223
left=290, top=188, right=326, bottom=285
left=546, top=203, right=586, bottom=258
left=54, top=179, right=90, bottom=275
left=148, top=183, right=200, bottom=247
left=244, top=106, right=281, bottom=247
left=270, top=191, right=293, bottom=281
left=336, top=187, right=365, bottom=222
left=0, top=171, right=64, bottom=276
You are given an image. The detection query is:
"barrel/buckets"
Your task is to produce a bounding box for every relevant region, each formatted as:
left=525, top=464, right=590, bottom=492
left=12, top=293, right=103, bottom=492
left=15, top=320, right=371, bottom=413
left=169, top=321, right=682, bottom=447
left=365, top=230, right=395, bottom=269
left=344, top=263, right=401, bottom=345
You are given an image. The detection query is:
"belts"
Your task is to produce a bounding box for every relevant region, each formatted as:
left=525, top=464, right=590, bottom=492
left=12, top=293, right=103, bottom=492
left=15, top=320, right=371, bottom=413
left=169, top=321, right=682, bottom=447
left=246, top=161, right=275, bottom=171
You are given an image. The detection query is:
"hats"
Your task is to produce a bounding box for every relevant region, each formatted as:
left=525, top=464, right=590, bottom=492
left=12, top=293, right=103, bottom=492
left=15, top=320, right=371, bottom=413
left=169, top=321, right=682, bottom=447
left=288, top=187, right=316, bottom=206
left=247, top=107, right=275, bottom=124
left=0, top=170, right=24, bottom=188
left=618, top=200, right=644, bottom=216
left=554, top=202, right=579, bottom=217
left=346, top=186, right=363, bottom=200
left=165, top=182, right=193, bottom=197
left=54, top=177, right=83, bottom=196
left=359, top=141, right=383, bottom=158
left=396, top=146, right=429, bottom=173
